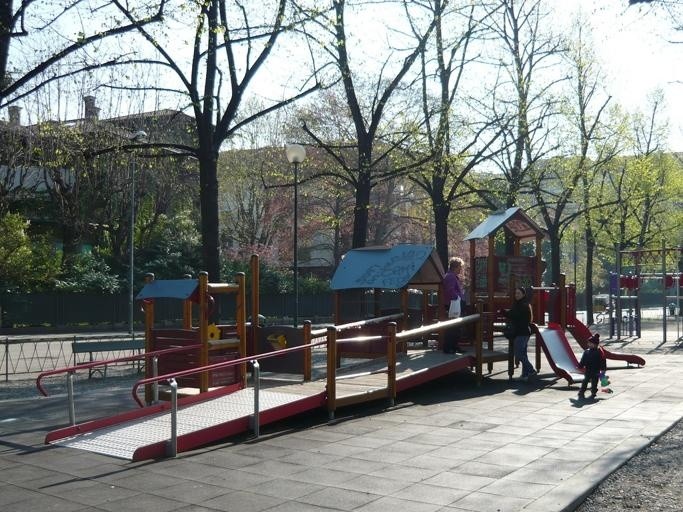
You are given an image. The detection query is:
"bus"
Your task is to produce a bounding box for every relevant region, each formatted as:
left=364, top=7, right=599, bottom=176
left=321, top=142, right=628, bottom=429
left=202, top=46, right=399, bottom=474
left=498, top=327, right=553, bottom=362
left=614, top=295, right=638, bottom=309
left=665, top=296, right=683, bottom=307
left=593, top=294, right=616, bottom=314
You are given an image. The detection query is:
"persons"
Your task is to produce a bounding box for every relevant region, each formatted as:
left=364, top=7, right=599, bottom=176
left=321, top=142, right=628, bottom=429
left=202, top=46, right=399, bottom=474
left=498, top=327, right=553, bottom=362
left=443, top=257, right=465, bottom=354
left=577, top=333, right=606, bottom=399
left=501, top=288, right=537, bottom=384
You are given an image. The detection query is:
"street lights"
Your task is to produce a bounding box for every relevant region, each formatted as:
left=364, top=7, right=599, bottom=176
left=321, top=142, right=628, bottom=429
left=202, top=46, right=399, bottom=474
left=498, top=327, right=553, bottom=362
left=287, top=144, right=307, bottom=329
left=127, top=130, right=147, bottom=334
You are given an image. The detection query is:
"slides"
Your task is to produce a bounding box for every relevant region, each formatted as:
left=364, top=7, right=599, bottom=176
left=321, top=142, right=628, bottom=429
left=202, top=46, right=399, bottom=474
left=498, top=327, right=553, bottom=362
left=531, top=324, right=586, bottom=384
left=567, top=318, right=645, bottom=365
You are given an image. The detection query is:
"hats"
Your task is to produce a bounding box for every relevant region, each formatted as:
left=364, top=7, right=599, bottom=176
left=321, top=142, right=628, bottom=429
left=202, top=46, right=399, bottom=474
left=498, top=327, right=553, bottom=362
left=589, top=334, right=601, bottom=345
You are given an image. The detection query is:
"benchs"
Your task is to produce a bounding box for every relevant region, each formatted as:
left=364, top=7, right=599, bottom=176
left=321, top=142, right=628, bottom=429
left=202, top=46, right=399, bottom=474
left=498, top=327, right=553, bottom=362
left=72, top=339, right=146, bottom=380
